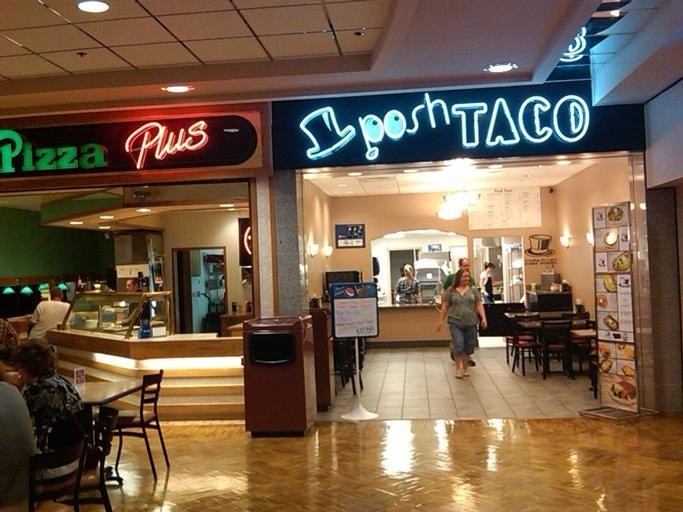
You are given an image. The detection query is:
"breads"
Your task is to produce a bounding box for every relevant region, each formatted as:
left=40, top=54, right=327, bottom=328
left=612, top=252, right=632, bottom=271
left=599, top=343, right=612, bottom=374
left=603, top=315, right=618, bottom=331
left=607, top=206, right=623, bottom=222
left=597, top=295, right=608, bottom=309
left=623, top=348, right=635, bottom=358
left=604, top=275, right=616, bottom=293
left=616, top=364, right=634, bottom=376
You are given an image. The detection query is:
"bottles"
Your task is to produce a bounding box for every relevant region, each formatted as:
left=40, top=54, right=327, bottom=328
left=231, top=298, right=237, bottom=313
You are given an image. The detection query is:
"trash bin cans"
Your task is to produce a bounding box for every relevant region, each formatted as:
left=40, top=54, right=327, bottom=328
left=243, top=308, right=336, bottom=438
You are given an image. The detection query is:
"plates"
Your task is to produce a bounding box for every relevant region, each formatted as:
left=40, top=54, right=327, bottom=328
left=608, top=208, right=625, bottom=224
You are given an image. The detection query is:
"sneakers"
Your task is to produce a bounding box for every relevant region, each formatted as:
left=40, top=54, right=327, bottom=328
left=449, top=350, right=477, bottom=379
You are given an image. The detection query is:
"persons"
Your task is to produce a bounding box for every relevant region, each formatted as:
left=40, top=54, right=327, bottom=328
left=14, top=336, right=86, bottom=485
left=0, top=379, right=45, bottom=512
left=28, top=286, right=75, bottom=340
left=441, top=257, right=477, bottom=367
left=435, top=268, right=487, bottom=380
left=479, top=261, right=496, bottom=303
left=114, top=278, right=151, bottom=326
left=0, top=318, right=25, bottom=393
left=393, top=264, right=421, bottom=305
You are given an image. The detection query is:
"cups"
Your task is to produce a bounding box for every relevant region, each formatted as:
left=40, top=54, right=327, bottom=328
left=239, top=305, right=244, bottom=313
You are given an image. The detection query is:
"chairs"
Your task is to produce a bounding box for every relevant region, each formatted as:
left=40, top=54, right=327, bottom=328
left=504, top=310, right=596, bottom=398
left=26, top=367, right=170, bottom=512
